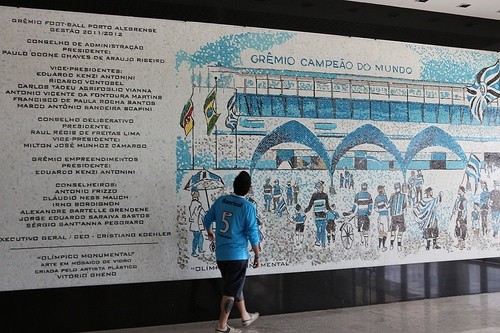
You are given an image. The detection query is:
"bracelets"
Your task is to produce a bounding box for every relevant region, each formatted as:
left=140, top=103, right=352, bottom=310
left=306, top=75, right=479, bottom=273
left=255, top=257, right=259, bottom=259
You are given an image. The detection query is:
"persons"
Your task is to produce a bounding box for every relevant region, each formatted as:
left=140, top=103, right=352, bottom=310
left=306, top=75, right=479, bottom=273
left=204, top=171, right=260, bottom=333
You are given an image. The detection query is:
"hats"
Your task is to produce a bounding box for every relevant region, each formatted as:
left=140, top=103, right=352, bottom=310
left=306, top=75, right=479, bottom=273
left=233, top=171, right=251, bottom=196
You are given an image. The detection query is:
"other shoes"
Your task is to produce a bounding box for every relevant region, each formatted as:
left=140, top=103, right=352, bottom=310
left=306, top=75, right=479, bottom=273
left=242, top=312, right=259, bottom=327
left=215, top=325, right=242, bottom=333
